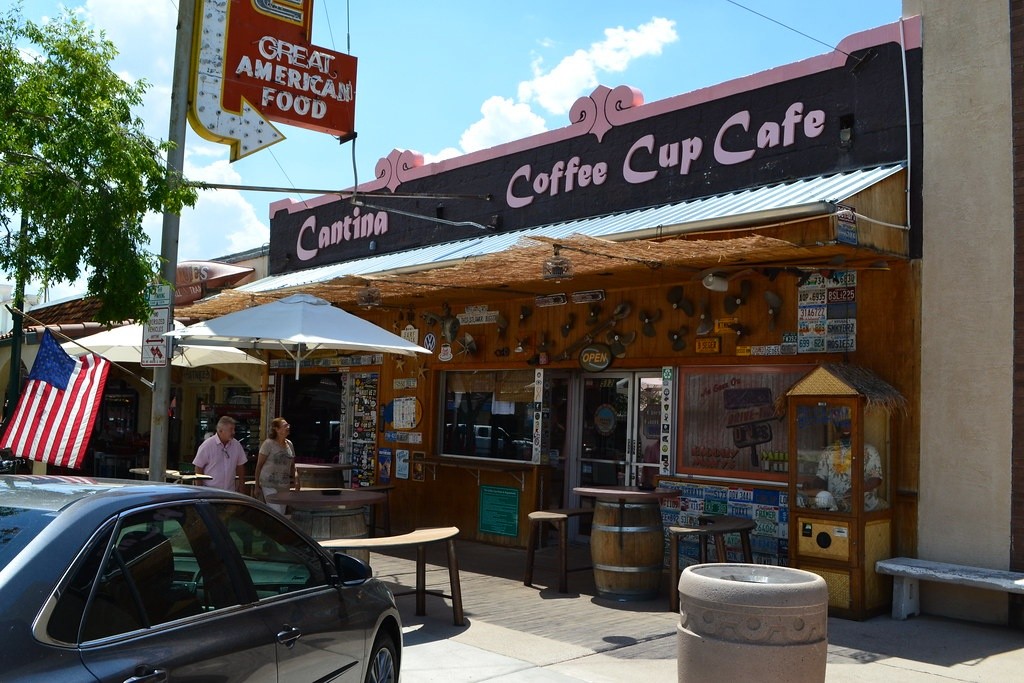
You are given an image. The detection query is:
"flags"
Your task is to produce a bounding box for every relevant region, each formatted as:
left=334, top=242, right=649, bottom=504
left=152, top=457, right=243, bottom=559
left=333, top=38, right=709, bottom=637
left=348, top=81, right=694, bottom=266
left=0, top=326, right=109, bottom=469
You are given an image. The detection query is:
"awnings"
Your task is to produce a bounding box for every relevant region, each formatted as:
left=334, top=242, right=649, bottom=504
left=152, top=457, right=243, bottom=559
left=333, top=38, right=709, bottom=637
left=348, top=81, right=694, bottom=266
left=170, top=164, right=904, bottom=318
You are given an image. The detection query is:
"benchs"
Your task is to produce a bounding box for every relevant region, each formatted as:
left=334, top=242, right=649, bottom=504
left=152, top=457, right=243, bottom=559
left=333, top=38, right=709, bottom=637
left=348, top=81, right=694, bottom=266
left=245, top=479, right=262, bottom=497
left=874, top=557, right=1024, bottom=622
left=289, top=484, right=397, bottom=538
left=314, top=526, right=465, bottom=627
left=669, top=514, right=758, bottom=611
left=523, top=508, right=595, bottom=594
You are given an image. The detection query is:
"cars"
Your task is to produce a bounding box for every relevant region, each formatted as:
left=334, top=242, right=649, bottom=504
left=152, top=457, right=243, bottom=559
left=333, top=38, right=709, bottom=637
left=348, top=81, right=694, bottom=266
left=0, top=474, right=405, bottom=683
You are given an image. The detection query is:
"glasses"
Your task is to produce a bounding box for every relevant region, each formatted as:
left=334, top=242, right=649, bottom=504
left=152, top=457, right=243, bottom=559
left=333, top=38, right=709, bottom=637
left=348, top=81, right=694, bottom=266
left=279, top=424, right=291, bottom=428
left=221, top=448, right=230, bottom=458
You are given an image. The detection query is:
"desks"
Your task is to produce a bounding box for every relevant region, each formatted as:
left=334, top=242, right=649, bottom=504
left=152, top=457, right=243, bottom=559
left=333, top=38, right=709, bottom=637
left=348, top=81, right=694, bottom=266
left=267, top=491, right=386, bottom=567
left=573, top=485, right=683, bottom=602
left=128, top=466, right=213, bottom=486
left=294, top=462, right=352, bottom=489
left=91, top=449, right=139, bottom=480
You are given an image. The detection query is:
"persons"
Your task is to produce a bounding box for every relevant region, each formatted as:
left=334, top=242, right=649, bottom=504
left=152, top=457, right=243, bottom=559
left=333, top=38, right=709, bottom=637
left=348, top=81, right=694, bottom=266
left=194, top=416, right=248, bottom=496
left=803, top=406, right=883, bottom=510
left=255, top=418, right=296, bottom=554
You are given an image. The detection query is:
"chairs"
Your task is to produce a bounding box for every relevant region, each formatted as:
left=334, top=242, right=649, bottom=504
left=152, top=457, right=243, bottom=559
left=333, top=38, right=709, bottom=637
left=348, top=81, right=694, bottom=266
left=102, top=531, right=203, bottom=628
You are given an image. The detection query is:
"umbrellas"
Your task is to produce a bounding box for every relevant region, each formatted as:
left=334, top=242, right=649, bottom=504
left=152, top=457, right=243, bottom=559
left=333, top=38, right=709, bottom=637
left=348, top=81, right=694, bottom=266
left=163, top=293, right=433, bottom=379
left=61, top=316, right=266, bottom=368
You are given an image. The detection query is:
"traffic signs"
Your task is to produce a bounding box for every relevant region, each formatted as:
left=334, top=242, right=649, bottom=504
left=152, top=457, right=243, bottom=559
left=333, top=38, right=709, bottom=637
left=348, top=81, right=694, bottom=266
left=138, top=306, right=171, bottom=368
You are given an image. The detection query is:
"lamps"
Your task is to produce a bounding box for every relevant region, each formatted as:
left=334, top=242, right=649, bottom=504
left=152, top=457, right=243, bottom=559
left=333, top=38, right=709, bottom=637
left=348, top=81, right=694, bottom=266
left=702, top=275, right=729, bottom=291
left=543, top=242, right=575, bottom=282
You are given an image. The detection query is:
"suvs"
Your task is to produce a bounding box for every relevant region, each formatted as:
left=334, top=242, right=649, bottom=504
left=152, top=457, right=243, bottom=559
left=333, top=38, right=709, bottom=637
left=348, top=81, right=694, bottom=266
left=446, top=419, right=533, bottom=457
